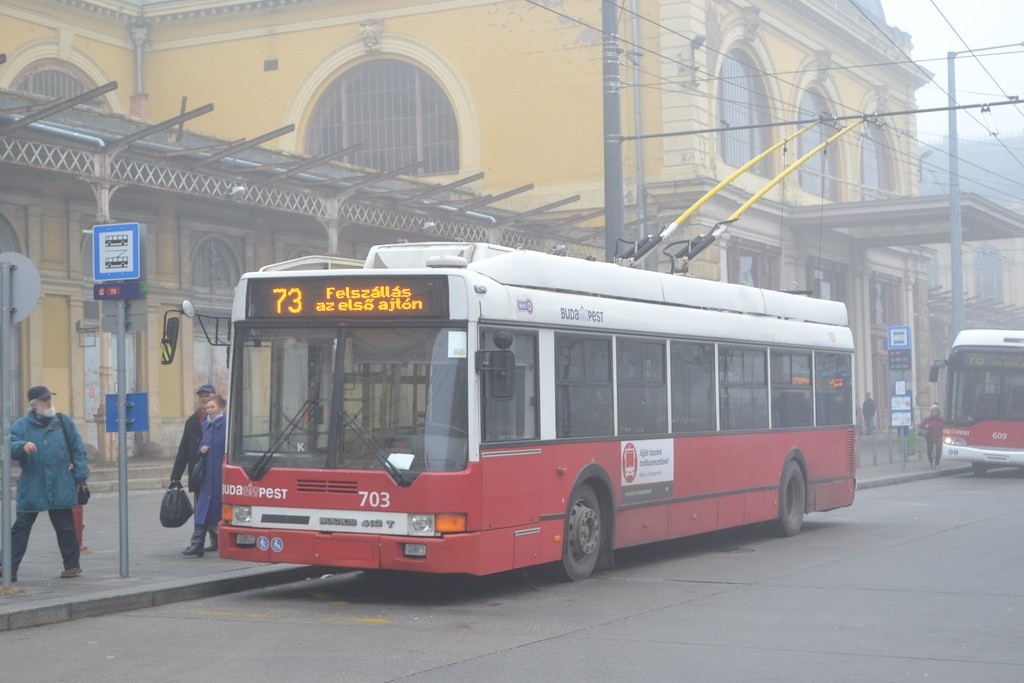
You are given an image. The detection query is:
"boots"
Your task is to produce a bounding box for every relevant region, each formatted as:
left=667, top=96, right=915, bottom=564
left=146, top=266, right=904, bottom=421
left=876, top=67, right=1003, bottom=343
left=204, top=528, right=217, bottom=551
left=183, top=524, right=208, bottom=557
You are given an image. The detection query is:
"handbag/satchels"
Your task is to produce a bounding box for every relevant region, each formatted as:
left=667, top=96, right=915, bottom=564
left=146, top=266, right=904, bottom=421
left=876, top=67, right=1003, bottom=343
left=918, top=427, right=929, bottom=437
left=160, top=482, right=194, bottom=528
left=190, top=454, right=206, bottom=492
left=78, top=482, right=91, bottom=505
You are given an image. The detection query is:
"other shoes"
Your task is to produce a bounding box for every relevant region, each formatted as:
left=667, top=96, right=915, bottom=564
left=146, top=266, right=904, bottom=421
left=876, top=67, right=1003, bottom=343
left=60, top=566, right=81, bottom=578
left=935, top=465, right=940, bottom=469
left=930, top=460, right=933, bottom=467
left=0, top=564, right=17, bottom=581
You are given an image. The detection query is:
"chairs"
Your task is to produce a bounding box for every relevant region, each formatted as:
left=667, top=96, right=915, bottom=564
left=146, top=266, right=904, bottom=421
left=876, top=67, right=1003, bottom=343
left=778, top=389, right=836, bottom=424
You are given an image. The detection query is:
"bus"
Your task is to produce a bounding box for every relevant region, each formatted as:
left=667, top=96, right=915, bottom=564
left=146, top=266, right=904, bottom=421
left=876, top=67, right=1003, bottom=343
left=157, top=114, right=858, bottom=582
left=928, top=328, right=1024, bottom=479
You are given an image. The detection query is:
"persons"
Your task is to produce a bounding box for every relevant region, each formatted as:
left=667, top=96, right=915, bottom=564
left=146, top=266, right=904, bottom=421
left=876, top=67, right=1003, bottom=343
left=862, top=392, right=876, bottom=435
left=170, top=385, right=216, bottom=514
left=180, top=394, right=227, bottom=558
left=0, top=386, right=91, bottom=580
left=920, top=405, right=945, bottom=467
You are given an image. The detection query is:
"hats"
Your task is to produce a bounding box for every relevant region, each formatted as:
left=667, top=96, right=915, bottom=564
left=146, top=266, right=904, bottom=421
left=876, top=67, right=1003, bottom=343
left=931, top=405, right=941, bottom=414
left=197, top=385, right=215, bottom=394
left=28, top=385, right=57, bottom=402
